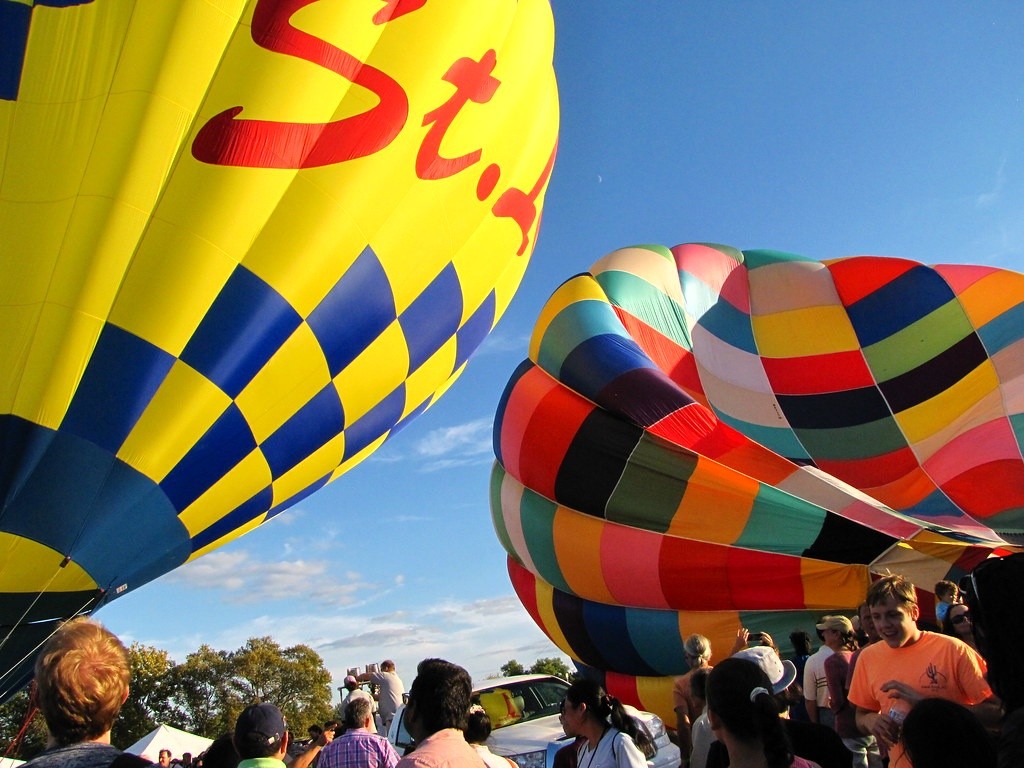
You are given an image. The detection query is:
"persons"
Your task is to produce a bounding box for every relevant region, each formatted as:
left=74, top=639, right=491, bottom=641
left=14, top=570, right=1024, bottom=768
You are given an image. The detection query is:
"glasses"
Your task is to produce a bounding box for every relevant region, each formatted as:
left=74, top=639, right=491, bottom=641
left=402, top=692, right=413, bottom=705
left=951, top=610, right=969, bottom=624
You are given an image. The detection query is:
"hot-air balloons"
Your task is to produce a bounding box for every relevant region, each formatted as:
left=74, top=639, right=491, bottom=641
left=0, top=0, right=559, bottom=768
left=491, top=243, right=1023, bottom=708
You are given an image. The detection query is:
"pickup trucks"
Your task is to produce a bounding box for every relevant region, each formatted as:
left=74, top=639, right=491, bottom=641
left=383, top=674, right=681, bottom=767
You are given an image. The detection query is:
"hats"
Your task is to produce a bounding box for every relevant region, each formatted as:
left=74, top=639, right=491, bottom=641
left=816, top=616, right=854, bottom=635
left=234, top=705, right=288, bottom=747
left=732, top=646, right=797, bottom=694
left=344, top=675, right=356, bottom=683
